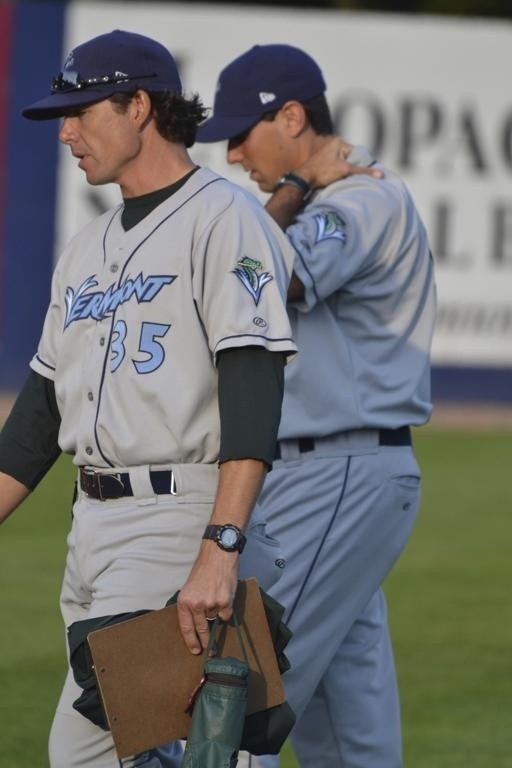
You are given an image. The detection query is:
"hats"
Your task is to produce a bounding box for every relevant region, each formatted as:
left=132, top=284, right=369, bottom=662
left=189, top=43, right=328, bottom=145
left=21, top=29, right=182, bottom=122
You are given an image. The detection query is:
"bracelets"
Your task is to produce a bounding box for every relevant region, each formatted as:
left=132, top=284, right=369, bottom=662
left=271, top=172, right=316, bottom=207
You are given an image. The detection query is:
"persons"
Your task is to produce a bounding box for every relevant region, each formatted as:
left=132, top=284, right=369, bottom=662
left=2, top=27, right=301, bottom=766
left=192, top=42, right=439, bottom=766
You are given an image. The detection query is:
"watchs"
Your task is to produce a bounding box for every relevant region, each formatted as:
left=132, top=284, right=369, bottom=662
left=201, top=520, right=247, bottom=557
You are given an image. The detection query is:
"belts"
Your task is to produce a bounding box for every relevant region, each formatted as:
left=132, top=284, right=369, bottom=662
left=80, top=470, right=177, bottom=503
left=275, top=425, right=411, bottom=457
